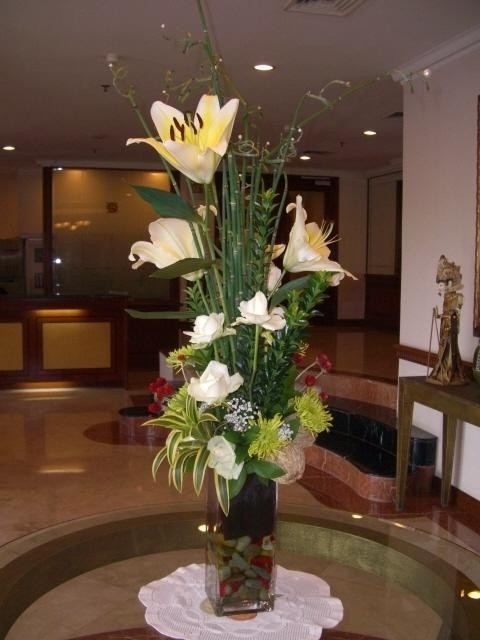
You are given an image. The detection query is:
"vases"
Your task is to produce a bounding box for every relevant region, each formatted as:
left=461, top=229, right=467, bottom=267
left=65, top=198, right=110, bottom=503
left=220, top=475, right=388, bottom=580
left=204, top=465, right=281, bottom=619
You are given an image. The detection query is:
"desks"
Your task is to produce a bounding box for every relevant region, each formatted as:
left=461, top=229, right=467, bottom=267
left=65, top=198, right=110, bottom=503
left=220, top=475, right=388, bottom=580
left=391, top=373, right=480, bottom=515
left=0, top=497, right=479, bottom=639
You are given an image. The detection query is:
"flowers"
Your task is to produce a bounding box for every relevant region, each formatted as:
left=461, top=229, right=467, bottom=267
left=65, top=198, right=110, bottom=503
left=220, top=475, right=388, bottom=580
left=99, top=4, right=428, bottom=517
